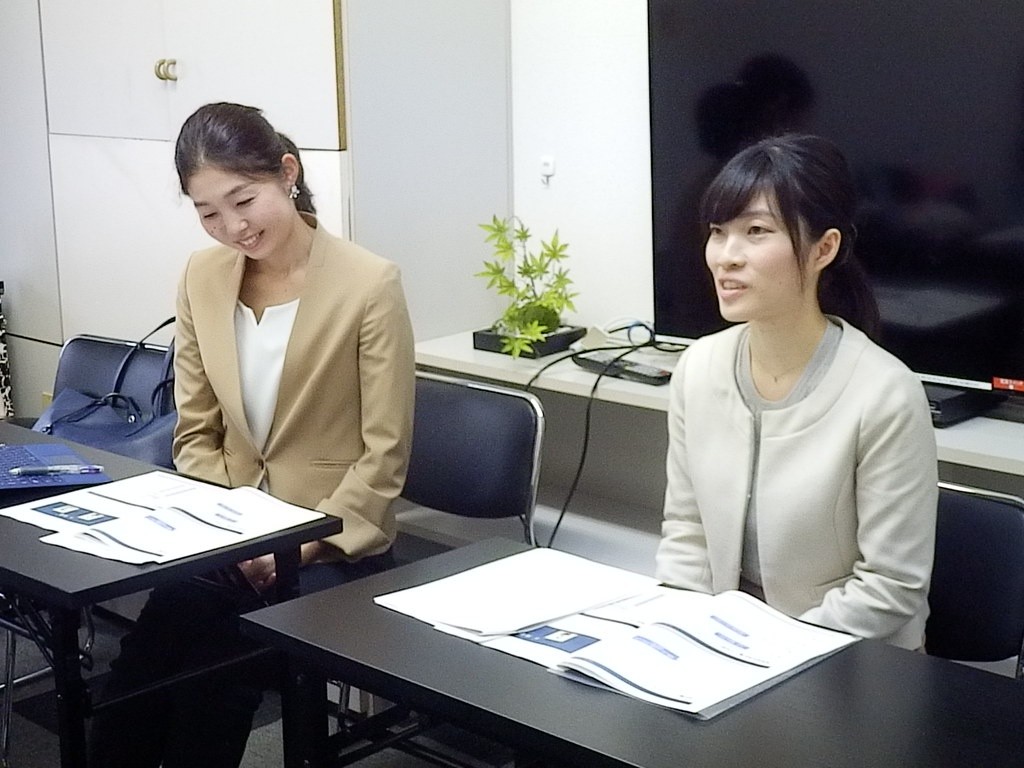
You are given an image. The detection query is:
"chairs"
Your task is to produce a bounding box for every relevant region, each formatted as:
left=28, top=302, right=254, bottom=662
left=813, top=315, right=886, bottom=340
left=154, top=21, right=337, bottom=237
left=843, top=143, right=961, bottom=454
left=0, top=335, right=177, bottom=690
left=923, top=484, right=1022, bottom=683
left=336, top=368, right=546, bottom=731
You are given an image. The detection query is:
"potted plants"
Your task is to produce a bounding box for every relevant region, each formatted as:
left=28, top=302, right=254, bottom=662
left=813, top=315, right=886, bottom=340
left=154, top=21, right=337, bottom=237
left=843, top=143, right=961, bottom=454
left=474, top=215, right=587, bottom=360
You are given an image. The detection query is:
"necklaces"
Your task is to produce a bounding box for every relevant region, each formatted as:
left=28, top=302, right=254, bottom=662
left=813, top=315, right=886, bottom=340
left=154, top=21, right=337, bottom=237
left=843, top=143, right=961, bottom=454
left=748, top=335, right=822, bottom=385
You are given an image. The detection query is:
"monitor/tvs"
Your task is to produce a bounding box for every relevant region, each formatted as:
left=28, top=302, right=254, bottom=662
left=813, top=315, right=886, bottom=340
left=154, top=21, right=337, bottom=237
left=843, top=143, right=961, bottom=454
left=646, top=0, right=1024, bottom=393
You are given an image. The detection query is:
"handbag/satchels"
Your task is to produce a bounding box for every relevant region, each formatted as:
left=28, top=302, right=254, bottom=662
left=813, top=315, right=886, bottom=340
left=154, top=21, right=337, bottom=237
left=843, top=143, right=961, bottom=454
left=31, top=315, right=176, bottom=470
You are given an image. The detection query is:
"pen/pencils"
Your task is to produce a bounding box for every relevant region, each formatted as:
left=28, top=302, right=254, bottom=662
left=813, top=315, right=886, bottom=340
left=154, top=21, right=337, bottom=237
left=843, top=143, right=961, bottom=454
left=8, top=464, right=105, bottom=477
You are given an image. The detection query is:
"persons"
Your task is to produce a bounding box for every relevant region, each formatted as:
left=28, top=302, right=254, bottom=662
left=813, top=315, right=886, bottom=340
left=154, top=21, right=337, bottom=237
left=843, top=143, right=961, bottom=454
left=653, top=131, right=938, bottom=653
left=85, top=103, right=415, bottom=768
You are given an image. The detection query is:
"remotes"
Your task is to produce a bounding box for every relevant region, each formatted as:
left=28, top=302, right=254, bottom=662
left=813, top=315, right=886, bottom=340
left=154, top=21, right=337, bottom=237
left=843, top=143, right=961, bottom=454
left=572, top=351, right=671, bottom=385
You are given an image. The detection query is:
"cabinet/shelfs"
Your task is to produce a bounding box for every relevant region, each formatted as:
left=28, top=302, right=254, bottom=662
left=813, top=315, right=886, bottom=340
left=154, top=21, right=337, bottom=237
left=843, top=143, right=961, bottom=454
left=396, top=324, right=1023, bottom=679
left=0, top=0, right=514, bottom=420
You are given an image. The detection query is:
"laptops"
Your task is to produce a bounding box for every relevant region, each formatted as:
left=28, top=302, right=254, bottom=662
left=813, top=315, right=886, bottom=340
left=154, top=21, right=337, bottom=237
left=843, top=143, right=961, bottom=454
left=0, top=443, right=113, bottom=500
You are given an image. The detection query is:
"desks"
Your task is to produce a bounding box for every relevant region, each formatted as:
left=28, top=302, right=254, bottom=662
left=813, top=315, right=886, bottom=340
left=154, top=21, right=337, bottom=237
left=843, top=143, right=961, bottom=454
left=0, top=419, right=342, bottom=768
left=240, top=534, right=1024, bottom=768
